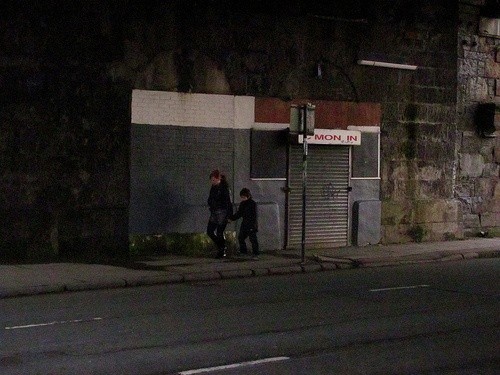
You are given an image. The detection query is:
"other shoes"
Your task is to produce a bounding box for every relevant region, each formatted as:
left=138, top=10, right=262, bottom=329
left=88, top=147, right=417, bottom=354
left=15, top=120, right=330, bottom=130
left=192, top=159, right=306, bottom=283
left=240, top=254, right=247, bottom=260
left=249, top=255, right=260, bottom=260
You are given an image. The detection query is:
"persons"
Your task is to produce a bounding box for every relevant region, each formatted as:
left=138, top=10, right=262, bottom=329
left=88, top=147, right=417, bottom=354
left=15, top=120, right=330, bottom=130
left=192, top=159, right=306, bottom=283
left=207, top=170, right=233, bottom=259
left=229, top=188, right=259, bottom=260
